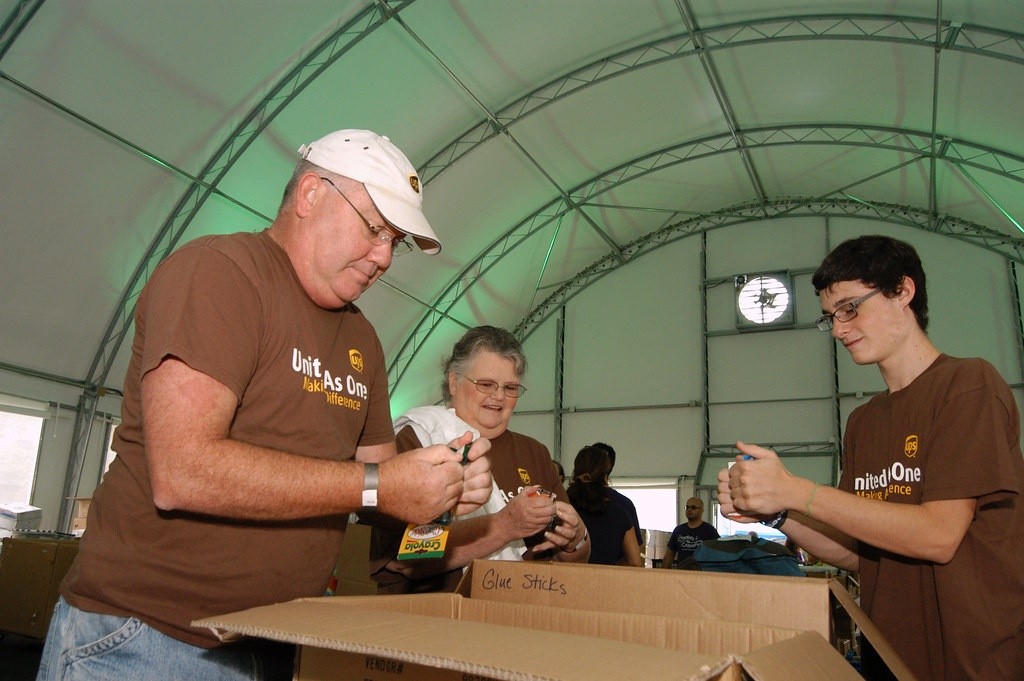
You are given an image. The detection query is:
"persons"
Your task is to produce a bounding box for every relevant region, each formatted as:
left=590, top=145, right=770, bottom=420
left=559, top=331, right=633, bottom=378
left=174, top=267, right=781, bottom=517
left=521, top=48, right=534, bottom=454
left=661, top=497, right=720, bottom=568
left=370, top=326, right=590, bottom=594
left=36, top=129, right=491, bottom=680
left=715, top=234, right=1024, bottom=681
left=551, top=442, right=645, bottom=566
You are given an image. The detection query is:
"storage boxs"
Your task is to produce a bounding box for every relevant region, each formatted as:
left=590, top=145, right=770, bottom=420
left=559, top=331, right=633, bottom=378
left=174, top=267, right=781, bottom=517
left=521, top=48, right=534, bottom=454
left=0, top=536, right=80, bottom=640
left=188, top=522, right=911, bottom=681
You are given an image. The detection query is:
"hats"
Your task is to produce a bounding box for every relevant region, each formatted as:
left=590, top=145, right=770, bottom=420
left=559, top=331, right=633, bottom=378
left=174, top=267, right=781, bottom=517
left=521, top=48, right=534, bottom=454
left=298, top=129, right=442, bottom=256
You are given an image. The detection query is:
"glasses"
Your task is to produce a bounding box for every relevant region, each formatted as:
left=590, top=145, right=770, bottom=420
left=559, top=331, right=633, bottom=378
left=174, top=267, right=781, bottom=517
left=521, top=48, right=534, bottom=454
left=684, top=505, right=701, bottom=511
left=455, top=371, right=528, bottom=398
left=319, top=176, right=414, bottom=257
left=816, top=287, right=883, bottom=332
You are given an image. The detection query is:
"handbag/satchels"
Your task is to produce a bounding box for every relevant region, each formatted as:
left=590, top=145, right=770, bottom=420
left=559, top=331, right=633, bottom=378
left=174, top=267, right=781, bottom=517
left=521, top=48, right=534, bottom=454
left=679, top=537, right=806, bottom=577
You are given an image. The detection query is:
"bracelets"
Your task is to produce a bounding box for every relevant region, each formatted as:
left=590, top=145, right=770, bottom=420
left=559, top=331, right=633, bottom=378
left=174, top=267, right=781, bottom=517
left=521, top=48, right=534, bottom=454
left=561, top=528, right=588, bottom=552
left=763, top=510, right=788, bottom=529
left=362, top=462, right=379, bottom=510
left=805, top=482, right=819, bottom=516
